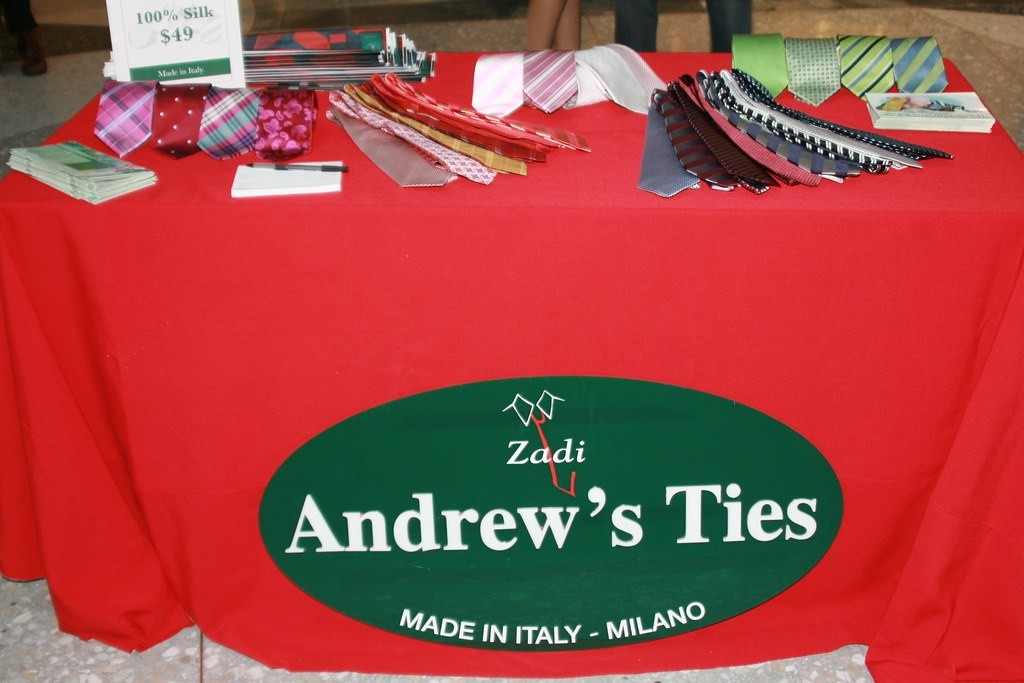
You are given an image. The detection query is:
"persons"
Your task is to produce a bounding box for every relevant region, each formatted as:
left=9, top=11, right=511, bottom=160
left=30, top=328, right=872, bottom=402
left=526, top=0, right=582, bottom=49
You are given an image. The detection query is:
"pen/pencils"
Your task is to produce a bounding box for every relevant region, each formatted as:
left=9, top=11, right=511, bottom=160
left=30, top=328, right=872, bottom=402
left=246, top=163, right=349, bottom=172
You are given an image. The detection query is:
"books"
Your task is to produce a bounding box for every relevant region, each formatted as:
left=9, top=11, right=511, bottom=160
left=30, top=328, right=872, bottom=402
left=242, top=26, right=436, bottom=89
left=865, top=92, right=996, bottom=133
left=231, top=161, right=343, bottom=198
left=5, top=140, right=160, bottom=206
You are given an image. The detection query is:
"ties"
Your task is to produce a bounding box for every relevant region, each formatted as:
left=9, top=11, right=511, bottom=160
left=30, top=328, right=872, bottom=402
left=471, top=42, right=669, bottom=116
left=638, top=33, right=953, bottom=198
left=95, top=81, right=320, bottom=163
left=328, top=71, right=591, bottom=186
